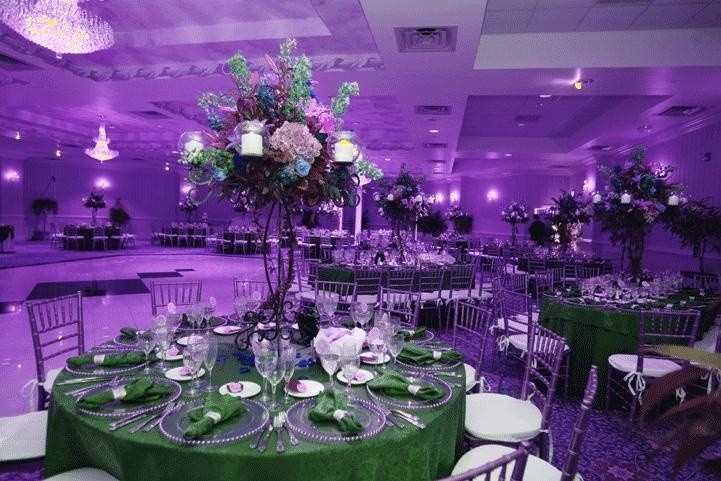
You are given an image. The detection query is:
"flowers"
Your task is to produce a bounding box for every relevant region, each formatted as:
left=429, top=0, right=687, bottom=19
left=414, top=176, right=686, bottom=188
left=80, top=186, right=108, bottom=226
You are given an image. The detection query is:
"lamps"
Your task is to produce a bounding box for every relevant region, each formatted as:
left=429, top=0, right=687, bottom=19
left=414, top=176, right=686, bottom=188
left=84, top=125, right=120, bottom=163
left=0, top=0, right=114, bottom=59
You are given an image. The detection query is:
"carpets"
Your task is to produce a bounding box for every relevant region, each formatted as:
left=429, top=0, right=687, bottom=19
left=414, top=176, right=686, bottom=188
left=0, top=239, right=303, bottom=269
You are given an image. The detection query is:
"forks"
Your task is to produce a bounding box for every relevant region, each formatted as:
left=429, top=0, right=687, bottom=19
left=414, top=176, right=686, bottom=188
left=279, top=409, right=298, bottom=446
left=128, top=403, right=176, bottom=433
left=144, top=400, right=186, bottom=432
left=273, top=414, right=286, bottom=453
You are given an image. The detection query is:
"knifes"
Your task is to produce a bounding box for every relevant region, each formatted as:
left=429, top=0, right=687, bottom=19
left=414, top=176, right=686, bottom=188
left=258, top=424, right=273, bottom=452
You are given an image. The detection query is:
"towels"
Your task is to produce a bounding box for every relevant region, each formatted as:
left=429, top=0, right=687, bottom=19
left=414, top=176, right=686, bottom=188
left=77, top=379, right=174, bottom=409
left=65, top=350, right=159, bottom=366
left=183, top=392, right=243, bottom=440
left=180, top=313, right=224, bottom=328
left=120, top=326, right=185, bottom=340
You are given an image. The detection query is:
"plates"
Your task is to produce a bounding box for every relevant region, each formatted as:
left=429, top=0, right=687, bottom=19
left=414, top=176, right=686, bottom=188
left=403, top=326, right=434, bottom=344
left=165, top=367, right=204, bottom=380
left=77, top=377, right=182, bottom=416
left=367, top=371, right=452, bottom=408
left=337, top=368, right=374, bottom=384
left=159, top=398, right=269, bottom=444
left=285, top=379, right=324, bottom=398
left=395, top=345, right=463, bottom=371
left=359, top=351, right=391, bottom=365
left=157, top=351, right=184, bottom=361
left=286, top=396, right=386, bottom=442
left=114, top=333, right=138, bottom=348
left=65, top=349, right=153, bottom=375
left=218, top=381, right=262, bottom=398
left=213, top=325, right=242, bottom=335
left=177, top=335, right=203, bottom=345
left=228, top=309, right=279, bottom=322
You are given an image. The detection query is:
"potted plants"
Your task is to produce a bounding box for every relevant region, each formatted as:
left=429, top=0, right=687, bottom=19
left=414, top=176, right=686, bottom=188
left=31, top=197, right=59, bottom=239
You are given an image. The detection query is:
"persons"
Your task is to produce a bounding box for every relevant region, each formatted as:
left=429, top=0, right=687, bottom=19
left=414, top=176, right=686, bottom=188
left=527, top=214, right=546, bottom=248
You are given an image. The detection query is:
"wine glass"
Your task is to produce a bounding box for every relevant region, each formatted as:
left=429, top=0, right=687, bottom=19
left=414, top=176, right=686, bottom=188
left=187, top=336, right=208, bottom=385
left=319, top=344, right=340, bottom=390
left=138, top=331, right=158, bottom=374
left=158, top=330, right=173, bottom=371
left=254, top=346, right=298, bottom=413
left=315, top=294, right=338, bottom=325
left=186, top=297, right=216, bottom=336
left=183, top=345, right=206, bottom=397
left=386, top=333, right=404, bottom=364
left=349, top=301, right=402, bottom=333
left=165, top=308, right=183, bottom=347
left=367, top=333, right=386, bottom=369
left=344, top=342, right=356, bottom=352
left=202, top=338, right=217, bottom=393
left=340, top=353, right=360, bottom=398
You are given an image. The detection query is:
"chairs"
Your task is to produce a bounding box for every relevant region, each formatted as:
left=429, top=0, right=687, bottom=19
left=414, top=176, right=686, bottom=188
left=0, top=409, right=49, bottom=473
left=26, top=290, right=85, bottom=411
left=432, top=238, right=605, bottom=296
left=233, top=277, right=279, bottom=300
left=295, top=259, right=478, bottom=336
left=41, top=467, right=119, bottom=481
left=150, top=279, right=202, bottom=315
